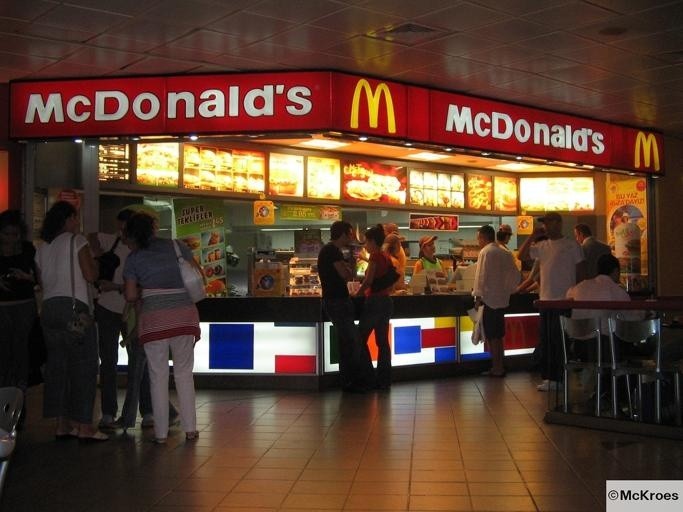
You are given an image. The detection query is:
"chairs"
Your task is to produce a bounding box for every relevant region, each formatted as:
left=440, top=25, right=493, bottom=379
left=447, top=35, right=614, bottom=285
left=608, top=316, right=680, bottom=424
left=559, top=315, right=634, bottom=420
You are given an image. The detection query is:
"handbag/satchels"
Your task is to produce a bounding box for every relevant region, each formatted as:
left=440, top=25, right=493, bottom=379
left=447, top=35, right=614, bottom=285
left=93, top=250, right=121, bottom=288
left=371, top=268, right=400, bottom=292
left=466, top=306, right=486, bottom=346
left=65, top=311, right=96, bottom=336
left=171, top=238, right=206, bottom=305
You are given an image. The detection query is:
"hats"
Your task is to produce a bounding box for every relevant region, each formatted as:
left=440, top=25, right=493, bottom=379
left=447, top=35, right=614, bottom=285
left=498, top=223, right=512, bottom=235
left=418, top=235, right=437, bottom=250
left=537, top=212, right=562, bottom=222
left=383, top=233, right=406, bottom=243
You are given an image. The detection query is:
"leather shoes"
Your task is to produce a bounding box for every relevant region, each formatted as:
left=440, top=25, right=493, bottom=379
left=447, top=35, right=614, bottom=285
left=481, top=370, right=501, bottom=377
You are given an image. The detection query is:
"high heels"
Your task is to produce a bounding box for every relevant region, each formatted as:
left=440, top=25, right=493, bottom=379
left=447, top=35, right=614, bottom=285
left=105, top=421, right=127, bottom=432
left=168, top=412, right=180, bottom=427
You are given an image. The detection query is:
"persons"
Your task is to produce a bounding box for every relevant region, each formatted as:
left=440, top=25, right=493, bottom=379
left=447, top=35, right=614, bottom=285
left=411, top=232, right=445, bottom=280
left=1, top=198, right=206, bottom=445
left=382, top=231, right=406, bottom=279
left=354, top=224, right=400, bottom=394
left=472, top=211, right=614, bottom=393
left=566, top=252, right=632, bottom=422
left=381, top=223, right=407, bottom=267
left=316, top=220, right=373, bottom=393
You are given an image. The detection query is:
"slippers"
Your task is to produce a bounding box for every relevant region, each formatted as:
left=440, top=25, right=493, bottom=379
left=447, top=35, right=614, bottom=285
left=76, top=429, right=109, bottom=443
left=55, top=425, right=78, bottom=439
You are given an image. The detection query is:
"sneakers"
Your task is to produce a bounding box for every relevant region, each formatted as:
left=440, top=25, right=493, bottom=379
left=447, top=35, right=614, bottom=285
left=186, top=431, right=199, bottom=440
left=141, top=413, right=155, bottom=428
left=151, top=437, right=167, bottom=443
left=96, top=415, right=113, bottom=426
left=536, top=382, right=552, bottom=391
